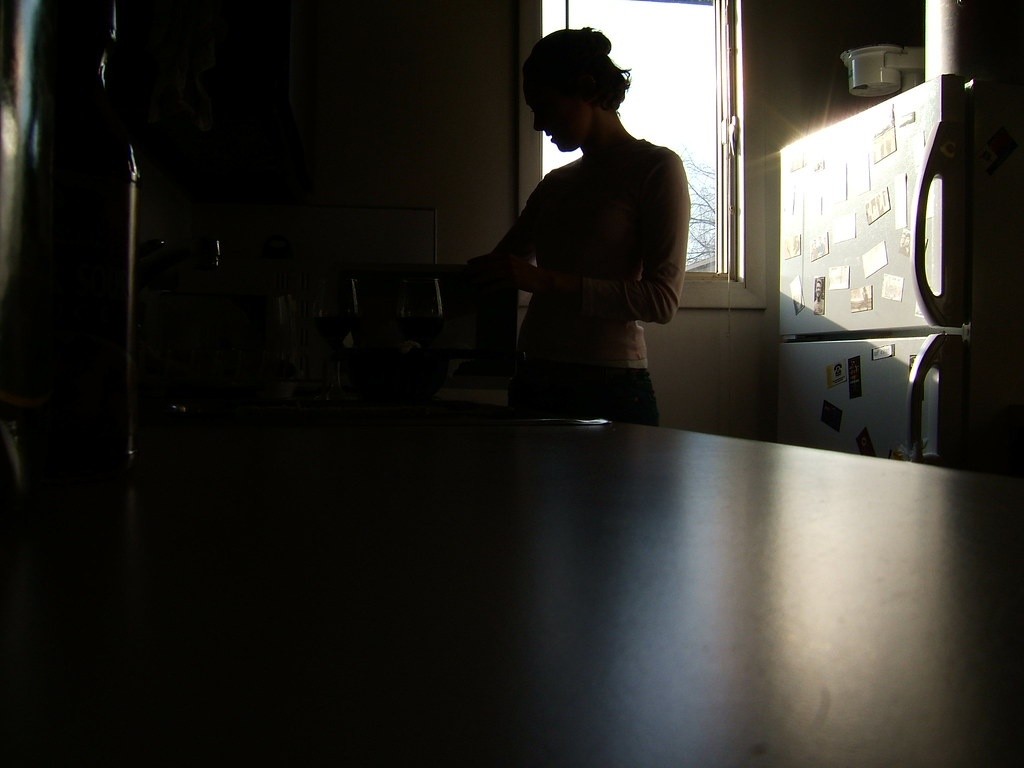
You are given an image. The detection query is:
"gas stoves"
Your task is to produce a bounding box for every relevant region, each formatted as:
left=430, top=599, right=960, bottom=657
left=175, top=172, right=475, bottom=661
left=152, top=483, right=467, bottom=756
left=332, top=346, right=521, bottom=403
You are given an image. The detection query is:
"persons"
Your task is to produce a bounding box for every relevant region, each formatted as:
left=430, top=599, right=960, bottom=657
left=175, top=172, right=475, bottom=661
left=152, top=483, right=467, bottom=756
left=464, top=30, right=691, bottom=426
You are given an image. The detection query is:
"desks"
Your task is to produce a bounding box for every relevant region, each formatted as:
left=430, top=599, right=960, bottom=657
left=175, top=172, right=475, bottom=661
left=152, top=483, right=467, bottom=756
left=0, top=417, right=1023, bottom=768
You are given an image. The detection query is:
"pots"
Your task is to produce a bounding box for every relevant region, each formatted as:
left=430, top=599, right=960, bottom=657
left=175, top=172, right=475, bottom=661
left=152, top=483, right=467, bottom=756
left=332, top=254, right=497, bottom=335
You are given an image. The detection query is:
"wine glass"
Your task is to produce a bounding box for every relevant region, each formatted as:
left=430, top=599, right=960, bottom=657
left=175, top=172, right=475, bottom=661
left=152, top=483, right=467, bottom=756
left=315, top=279, right=355, bottom=401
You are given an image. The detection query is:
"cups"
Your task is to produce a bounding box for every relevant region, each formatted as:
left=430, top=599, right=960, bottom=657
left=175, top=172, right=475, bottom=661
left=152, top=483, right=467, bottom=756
left=396, top=276, right=444, bottom=343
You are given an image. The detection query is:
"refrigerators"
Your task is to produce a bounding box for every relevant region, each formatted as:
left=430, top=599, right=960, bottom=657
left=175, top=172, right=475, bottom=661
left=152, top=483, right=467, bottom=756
left=775, top=75, right=1023, bottom=463
